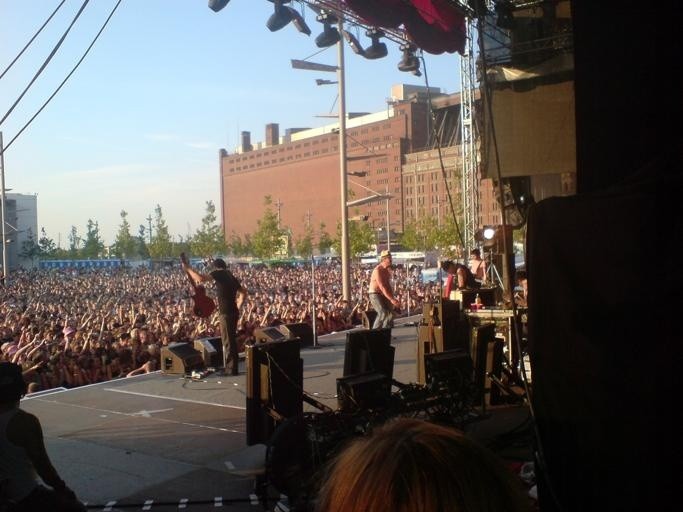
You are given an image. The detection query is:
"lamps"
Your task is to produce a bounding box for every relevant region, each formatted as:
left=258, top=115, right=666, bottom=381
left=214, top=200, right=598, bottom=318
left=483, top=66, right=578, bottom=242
left=209, top=0, right=420, bottom=72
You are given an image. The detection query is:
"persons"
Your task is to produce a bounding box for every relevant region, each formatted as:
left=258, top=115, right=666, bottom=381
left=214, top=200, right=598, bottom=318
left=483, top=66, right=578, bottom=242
left=181, top=257, right=248, bottom=377
left=314, top=417, right=529, bottom=512
left=513, top=271, right=528, bottom=305
left=1, top=360, right=87, bottom=511
left=470, top=249, right=487, bottom=283
left=1, top=262, right=221, bottom=392
left=439, top=260, right=478, bottom=303
left=367, top=249, right=400, bottom=342
left=230, top=263, right=443, bottom=354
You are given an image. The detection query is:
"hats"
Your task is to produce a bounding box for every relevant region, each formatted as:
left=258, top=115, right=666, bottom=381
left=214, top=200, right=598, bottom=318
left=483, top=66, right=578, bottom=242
left=377, top=249, right=398, bottom=261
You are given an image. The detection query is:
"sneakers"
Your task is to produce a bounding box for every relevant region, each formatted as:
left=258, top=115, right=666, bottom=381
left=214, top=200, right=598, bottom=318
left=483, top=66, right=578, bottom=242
left=217, top=368, right=238, bottom=376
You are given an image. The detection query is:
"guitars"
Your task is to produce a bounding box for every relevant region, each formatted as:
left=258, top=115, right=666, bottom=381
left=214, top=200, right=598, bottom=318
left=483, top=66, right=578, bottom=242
left=179, top=252, right=215, bottom=318
left=355, top=280, right=367, bottom=319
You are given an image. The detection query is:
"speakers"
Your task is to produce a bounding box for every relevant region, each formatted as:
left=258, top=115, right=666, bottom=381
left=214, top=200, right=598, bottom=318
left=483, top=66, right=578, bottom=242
left=482, top=224, right=515, bottom=307
left=194, top=336, right=223, bottom=368
left=280, top=322, right=317, bottom=348
left=362, top=310, right=394, bottom=329
left=255, top=326, right=284, bottom=346
left=160, top=341, right=205, bottom=375
left=418, top=300, right=472, bottom=385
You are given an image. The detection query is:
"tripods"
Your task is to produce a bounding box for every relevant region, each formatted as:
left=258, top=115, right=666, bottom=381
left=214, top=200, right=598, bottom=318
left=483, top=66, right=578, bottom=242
left=480, top=250, right=503, bottom=289
